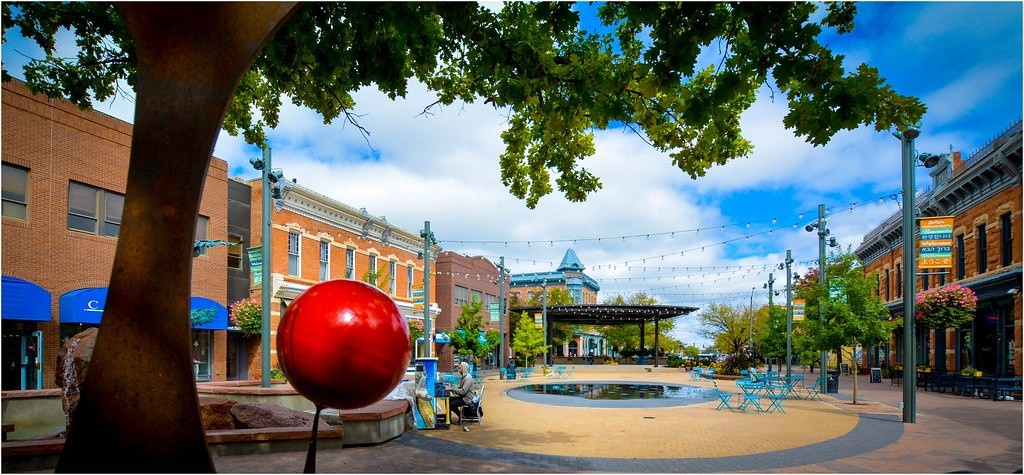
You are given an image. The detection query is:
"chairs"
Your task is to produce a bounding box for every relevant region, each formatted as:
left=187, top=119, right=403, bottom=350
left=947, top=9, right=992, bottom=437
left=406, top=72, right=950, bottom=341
left=501, top=368, right=533, bottom=382
left=442, top=365, right=487, bottom=389
left=460, top=385, right=485, bottom=427
left=568, top=366, right=574, bottom=378
left=687, top=369, right=698, bottom=381
left=713, top=370, right=822, bottom=414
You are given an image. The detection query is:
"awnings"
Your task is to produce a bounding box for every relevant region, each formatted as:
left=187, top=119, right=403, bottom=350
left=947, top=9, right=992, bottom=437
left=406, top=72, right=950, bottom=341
left=456, top=329, right=488, bottom=345
left=1, top=275, right=52, bottom=321
left=59, top=287, right=109, bottom=323
left=416, top=333, right=452, bottom=343
left=885, top=262, right=1023, bottom=316
left=190, top=296, right=228, bottom=331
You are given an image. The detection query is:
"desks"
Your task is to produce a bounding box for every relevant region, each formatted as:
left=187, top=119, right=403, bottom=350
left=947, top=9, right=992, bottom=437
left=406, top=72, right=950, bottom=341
left=769, top=375, right=805, bottom=400
left=555, top=366, right=566, bottom=378
left=734, top=380, right=790, bottom=410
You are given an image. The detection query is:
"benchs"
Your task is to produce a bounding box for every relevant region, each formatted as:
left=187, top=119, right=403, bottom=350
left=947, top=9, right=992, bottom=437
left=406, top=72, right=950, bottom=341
left=891, top=370, right=1023, bottom=401
left=340, top=400, right=408, bottom=445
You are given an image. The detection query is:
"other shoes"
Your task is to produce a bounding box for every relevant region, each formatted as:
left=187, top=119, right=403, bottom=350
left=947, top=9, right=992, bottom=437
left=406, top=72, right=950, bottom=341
left=457, top=417, right=467, bottom=423
left=440, top=419, right=453, bottom=424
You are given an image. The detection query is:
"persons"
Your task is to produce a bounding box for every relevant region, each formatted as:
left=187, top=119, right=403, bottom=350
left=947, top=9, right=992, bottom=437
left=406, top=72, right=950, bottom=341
left=755, top=364, right=767, bottom=378
left=444, top=362, right=474, bottom=423
